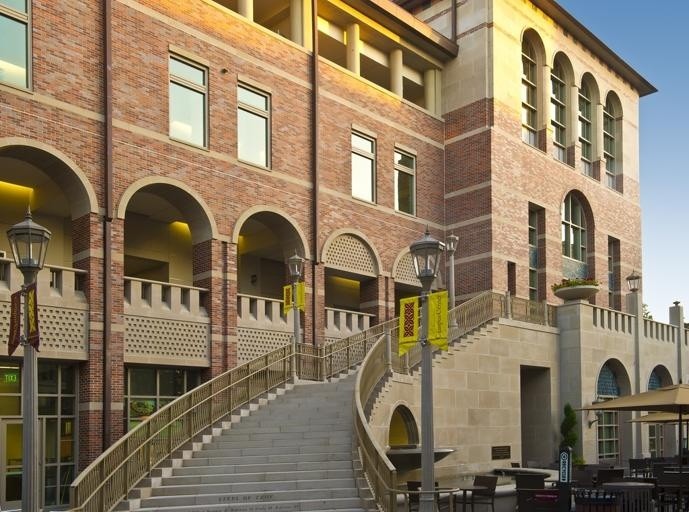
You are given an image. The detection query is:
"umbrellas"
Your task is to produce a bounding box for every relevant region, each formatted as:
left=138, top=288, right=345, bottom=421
left=625, top=411, right=689, bottom=474
left=573, top=378, right=689, bottom=511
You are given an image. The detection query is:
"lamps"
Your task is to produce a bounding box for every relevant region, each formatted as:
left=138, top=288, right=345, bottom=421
left=625, top=269, right=643, bottom=292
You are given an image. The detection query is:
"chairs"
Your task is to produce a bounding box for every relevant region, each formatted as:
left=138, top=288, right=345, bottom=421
left=405, top=450, right=689, bottom=510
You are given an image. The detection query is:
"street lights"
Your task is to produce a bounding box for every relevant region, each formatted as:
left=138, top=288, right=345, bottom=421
left=5, top=202, right=50, bottom=512
left=410, top=225, right=441, bottom=512
left=444, top=231, right=460, bottom=337
left=626, top=270, right=642, bottom=291
left=284, top=249, right=305, bottom=381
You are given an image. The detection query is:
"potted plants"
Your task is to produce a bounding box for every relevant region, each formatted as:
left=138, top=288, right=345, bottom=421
left=549, top=272, right=601, bottom=304
